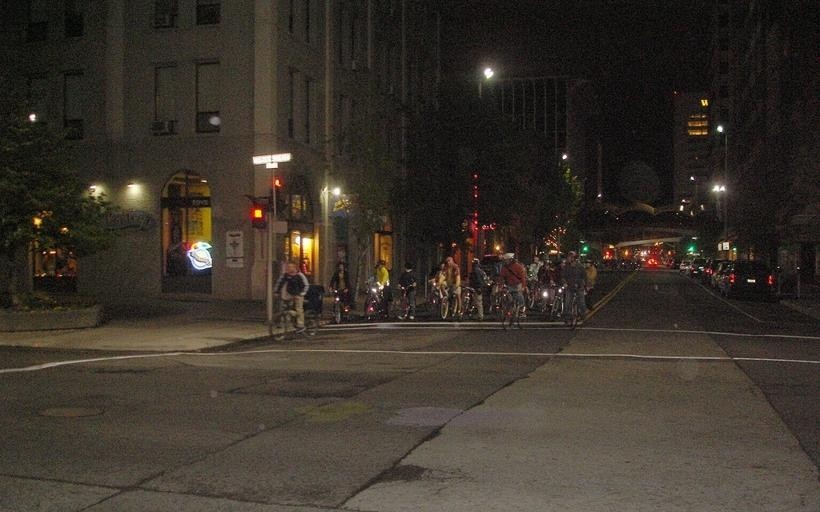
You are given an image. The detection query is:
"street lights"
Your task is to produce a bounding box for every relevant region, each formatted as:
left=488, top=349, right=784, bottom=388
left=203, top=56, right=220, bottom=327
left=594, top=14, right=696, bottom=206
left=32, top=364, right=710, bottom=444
left=475, top=66, right=495, bottom=96
left=715, top=123, right=731, bottom=260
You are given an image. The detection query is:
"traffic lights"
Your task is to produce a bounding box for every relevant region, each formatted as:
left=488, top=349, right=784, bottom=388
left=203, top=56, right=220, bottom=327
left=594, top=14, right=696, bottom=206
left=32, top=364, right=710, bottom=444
left=244, top=192, right=269, bottom=230
left=271, top=172, right=289, bottom=216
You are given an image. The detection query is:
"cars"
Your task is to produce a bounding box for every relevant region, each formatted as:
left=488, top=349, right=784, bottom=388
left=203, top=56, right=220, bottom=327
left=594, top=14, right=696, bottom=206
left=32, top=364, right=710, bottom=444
left=648, top=258, right=658, bottom=267
left=478, top=254, right=501, bottom=276
left=661, top=257, right=776, bottom=300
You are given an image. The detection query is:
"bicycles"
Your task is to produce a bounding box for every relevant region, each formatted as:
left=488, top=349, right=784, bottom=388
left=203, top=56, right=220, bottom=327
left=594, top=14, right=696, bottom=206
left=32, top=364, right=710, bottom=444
left=601, top=258, right=643, bottom=272
left=363, top=285, right=392, bottom=321
left=424, top=278, right=590, bottom=332
left=389, top=284, right=416, bottom=320
left=328, top=289, right=358, bottom=325
left=268, top=293, right=319, bottom=343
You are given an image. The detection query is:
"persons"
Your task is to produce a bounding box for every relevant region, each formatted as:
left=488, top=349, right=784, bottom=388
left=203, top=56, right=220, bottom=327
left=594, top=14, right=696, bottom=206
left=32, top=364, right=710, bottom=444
left=429, top=250, right=597, bottom=321
left=398, top=263, right=416, bottom=320
left=274, top=261, right=309, bottom=334
left=57, top=254, right=77, bottom=298
left=329, top=261, right=351, bottom=313
left=362, top=259, right=392, bottom=319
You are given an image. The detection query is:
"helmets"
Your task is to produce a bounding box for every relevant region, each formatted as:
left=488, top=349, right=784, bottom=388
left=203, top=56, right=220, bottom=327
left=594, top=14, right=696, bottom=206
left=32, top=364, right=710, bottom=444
left=503, top=252, right=514, bottom=259
